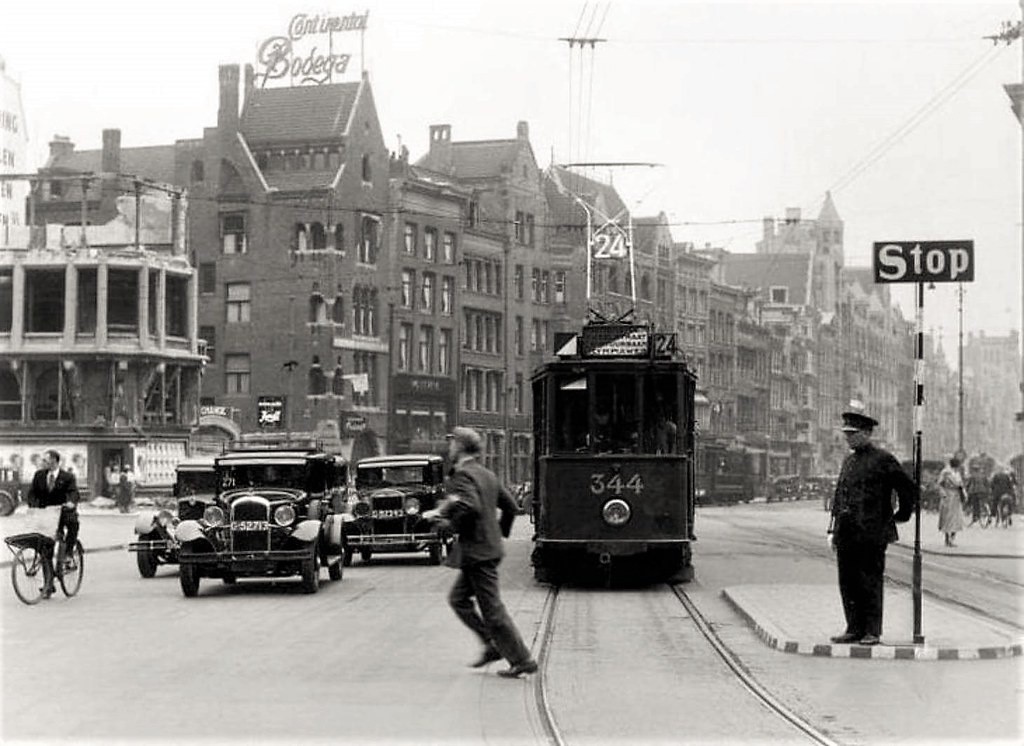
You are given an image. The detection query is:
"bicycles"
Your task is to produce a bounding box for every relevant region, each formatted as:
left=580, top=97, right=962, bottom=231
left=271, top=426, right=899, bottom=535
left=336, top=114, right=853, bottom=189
left=966, top=482, right=1015, bottom=529
left=4, top=505, right=85, bottom=606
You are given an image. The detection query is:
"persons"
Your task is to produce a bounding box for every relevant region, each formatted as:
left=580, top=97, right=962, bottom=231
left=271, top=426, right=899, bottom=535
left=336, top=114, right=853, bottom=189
left=648, top=412, right=677, bottom=455
left=420, top=424, right=538, bottom=680
left=826, top=413, right=915, bottom=647
left=935, top=459, right=1016, bottom=548
left=104, top=463, right=137, bottom=513
left=590, top=403, right=634, bottom=452
left=28, top=449, right=80, bottom=595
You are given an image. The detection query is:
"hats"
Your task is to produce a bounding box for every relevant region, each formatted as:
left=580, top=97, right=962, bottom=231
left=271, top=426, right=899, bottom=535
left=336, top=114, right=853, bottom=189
left=446, top=425, right=482, bottom=453
left=840, top=412, right=879, bottom=433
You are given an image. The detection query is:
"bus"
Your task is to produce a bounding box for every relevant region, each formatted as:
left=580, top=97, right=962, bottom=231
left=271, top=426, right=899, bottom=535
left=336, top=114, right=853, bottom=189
left=526, top=304, right=700, bottom=585
left=692, top=430, right=839, bottom=507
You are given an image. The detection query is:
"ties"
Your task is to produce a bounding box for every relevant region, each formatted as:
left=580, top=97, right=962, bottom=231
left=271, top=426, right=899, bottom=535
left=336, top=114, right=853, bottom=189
left=48, top=475, right=55, bottom=491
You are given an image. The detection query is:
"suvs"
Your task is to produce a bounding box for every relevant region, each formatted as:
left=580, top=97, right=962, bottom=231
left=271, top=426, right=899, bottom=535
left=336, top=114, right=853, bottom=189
left=344, top=453, right=458, bottom=565
left=902, top=458, right=948, bottom=511
left=127, top=484, right=181, bottom=579
left=174, top=430, right=355, bottom=597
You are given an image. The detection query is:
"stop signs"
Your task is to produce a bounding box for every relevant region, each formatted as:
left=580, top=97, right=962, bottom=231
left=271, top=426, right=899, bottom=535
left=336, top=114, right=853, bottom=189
left=873, top=239, right=974, bottom=284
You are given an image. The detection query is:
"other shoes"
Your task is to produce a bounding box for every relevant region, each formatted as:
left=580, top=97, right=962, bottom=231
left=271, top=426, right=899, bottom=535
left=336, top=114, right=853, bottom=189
left=497, top=660, right=538, bottom=678
left=42, top=585, right=57, bottom=599
left=830, top=632, right=857, bottom=643
left=470, top=646, right=502, bottom=668
left=859, top=634, right=879, bottom=645
left=66, top=556, right=79, bottom=571
